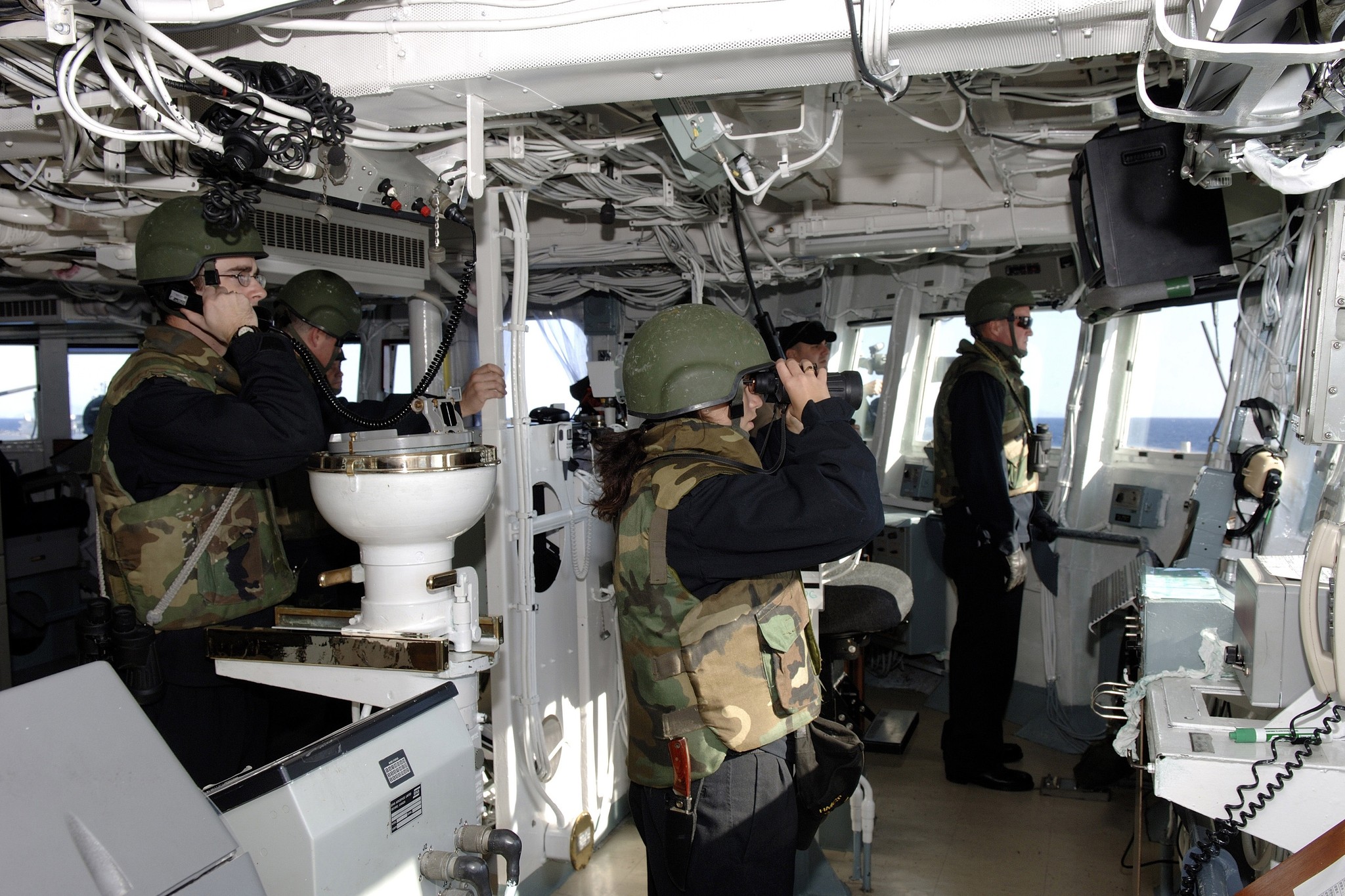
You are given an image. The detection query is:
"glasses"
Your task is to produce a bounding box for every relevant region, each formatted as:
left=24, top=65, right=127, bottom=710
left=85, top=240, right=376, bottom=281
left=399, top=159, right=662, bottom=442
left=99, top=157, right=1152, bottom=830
left=198, top=271, right=266, bottom=288
left=1014, top=316, right=1033, bottom=329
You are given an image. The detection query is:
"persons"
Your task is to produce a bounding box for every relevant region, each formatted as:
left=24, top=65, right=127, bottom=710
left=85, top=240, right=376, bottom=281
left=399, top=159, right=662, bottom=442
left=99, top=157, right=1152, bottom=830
left=78, top=195, right=508, bottom=793
left=779, top=320, right=862, bottom=441
left=590, top=294, right=886, bottom=896
left=925, top=276, right=1061, bottom=793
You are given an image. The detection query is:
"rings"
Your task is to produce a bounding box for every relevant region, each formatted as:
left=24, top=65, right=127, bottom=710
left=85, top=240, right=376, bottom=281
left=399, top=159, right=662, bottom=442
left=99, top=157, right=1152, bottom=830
left=802, top=366, right=815, bottom=374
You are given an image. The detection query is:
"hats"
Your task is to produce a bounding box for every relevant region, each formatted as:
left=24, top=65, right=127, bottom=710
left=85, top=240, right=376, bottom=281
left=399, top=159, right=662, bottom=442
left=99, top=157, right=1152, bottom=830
left=775, top=321, right=837, bottom=352
left=794, top=717, right=864, bottom=848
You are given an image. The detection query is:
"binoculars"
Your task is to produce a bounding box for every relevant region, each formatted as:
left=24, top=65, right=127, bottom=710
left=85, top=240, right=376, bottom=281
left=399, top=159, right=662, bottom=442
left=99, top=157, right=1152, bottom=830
left=1031, top=423, right=1053, bottom=474
left=751, top=370, right=864, bottom=413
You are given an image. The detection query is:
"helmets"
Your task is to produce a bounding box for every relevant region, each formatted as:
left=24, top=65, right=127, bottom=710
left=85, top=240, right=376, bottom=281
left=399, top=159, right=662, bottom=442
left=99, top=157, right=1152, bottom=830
left=965, top=276, right=1036, bottom=325
left=275, top=269, right=362, bottom=343
left=135, top=196, right=269, bottom=284
left=335, top=348, right=348, bottom=361
left=622, top=303, right=776, bottom=420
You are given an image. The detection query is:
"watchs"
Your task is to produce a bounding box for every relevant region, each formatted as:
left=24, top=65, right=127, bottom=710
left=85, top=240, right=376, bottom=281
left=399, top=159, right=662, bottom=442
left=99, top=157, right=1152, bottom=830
left=228, top=325, right=262, bottom=346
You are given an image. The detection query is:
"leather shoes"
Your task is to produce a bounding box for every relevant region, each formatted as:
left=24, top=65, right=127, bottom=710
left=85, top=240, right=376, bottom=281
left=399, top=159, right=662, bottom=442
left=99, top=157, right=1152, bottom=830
left=944, top=762, right=1035, bottom=792
left=999, top=742, right=1024, bottom=764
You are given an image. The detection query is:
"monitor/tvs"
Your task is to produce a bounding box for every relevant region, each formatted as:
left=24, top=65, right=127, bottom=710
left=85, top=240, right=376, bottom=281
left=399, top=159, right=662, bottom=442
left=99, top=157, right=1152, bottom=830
left=1064, top=122, right=1236, bottom=292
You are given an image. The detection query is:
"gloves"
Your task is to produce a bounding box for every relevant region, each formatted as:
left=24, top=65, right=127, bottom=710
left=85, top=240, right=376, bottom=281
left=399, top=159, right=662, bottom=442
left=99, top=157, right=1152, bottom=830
left=996, top=531, right=1029, bottom=592
left=1029, top=509, right=1059, bottom=543
